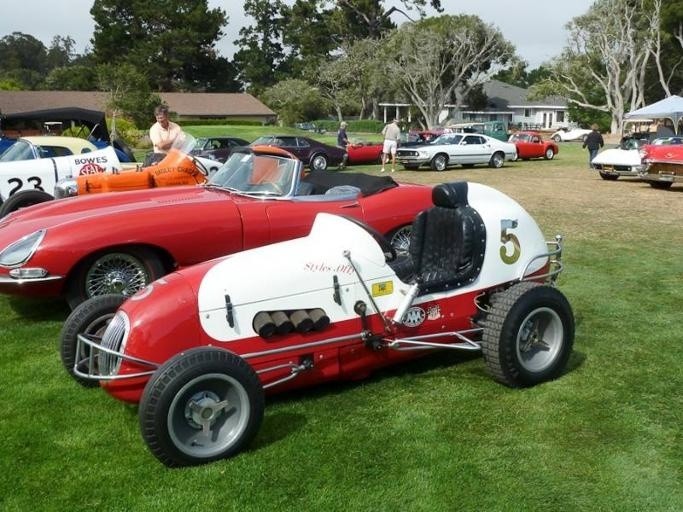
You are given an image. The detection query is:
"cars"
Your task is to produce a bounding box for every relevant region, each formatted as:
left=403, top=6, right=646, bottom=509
left=503, top=131, right=558, bottom=162
left=296, top=123, right=325, bottom=135
left=188, top=137, right=250, bottom=164
left=407, top=121, right=508, bottom=144
left=636, top=137, right=682, bottom=189
left=0, top=135, right=142, bottom=171
left=395, top=133, right=517, bottom=171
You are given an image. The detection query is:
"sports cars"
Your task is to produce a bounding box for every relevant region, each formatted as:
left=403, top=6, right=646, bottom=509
left=590, top=138, right=648, bottom=180
left=550, top=126, right=592, bottom=142
left=0, top=152, right=434, bottom=313
left=345, top=138, right=392, bottom=165
left=208, top=135, right=345, bottom=171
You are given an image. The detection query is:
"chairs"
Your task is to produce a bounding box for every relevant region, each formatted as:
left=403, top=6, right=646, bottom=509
left=389, top=184, right=473, bottom=297
left=325, top=185, right=360, bottom=196
left=283, top=182, right=316, bottom=196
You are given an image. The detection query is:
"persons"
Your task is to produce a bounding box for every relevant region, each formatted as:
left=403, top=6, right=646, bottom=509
left=149, top=105, right=186, bottom=163
left=582, top=123, right=604, bottom=170
left=337, top=121, right=352, bottom=169
left=380, top=119, right=401, bottom=173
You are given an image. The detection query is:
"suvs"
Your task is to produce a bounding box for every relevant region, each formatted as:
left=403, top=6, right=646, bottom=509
left=60, top=180, right=574, bottom=470
left=0, top=146, right=304, bottom=218
